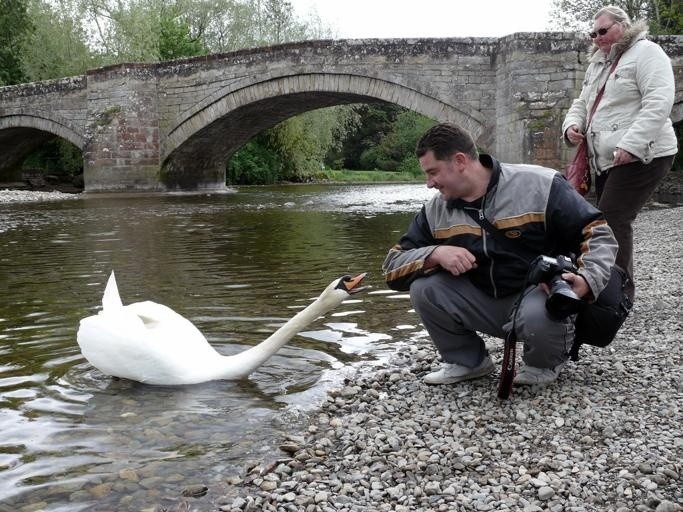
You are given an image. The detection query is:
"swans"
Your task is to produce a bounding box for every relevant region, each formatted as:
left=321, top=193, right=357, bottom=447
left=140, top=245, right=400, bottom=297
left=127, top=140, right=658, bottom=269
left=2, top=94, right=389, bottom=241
left=76, top=268, right=368, bottom=387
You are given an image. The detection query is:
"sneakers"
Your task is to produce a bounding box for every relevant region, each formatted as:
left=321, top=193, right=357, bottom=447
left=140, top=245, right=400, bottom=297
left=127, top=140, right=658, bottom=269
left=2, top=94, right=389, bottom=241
left=513, top=361, right=566, bottom=386
left=424, top=348, right=496, bottom=386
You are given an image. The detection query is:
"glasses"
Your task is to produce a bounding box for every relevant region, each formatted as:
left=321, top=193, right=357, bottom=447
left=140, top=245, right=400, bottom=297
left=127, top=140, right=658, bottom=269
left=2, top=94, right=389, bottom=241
left=590, top=22, right=616, bottom=38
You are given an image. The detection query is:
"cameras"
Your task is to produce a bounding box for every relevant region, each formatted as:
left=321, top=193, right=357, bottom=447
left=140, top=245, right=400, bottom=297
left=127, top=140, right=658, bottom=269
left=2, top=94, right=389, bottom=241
left=528, top=255, right=585, bottom=321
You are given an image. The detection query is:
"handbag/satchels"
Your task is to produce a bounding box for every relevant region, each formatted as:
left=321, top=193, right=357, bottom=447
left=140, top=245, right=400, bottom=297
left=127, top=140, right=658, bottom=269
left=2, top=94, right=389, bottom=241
left=566, top=138, right=591, bottom=197
left=573, top=265, right=634, bottom=348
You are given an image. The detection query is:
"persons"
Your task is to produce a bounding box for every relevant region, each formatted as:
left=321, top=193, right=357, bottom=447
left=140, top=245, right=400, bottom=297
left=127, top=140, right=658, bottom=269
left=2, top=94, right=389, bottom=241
left=381, top=121, right=621, bottom=384
left=560, top=6, right=678, bottom=318
